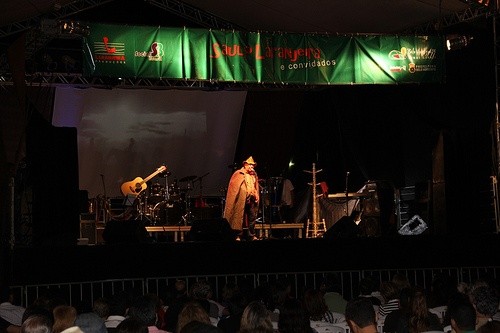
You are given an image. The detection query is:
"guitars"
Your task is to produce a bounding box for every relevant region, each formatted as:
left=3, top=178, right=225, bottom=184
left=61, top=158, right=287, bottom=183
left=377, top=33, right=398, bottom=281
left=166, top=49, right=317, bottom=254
left=121, top=165, right=166, bottom=196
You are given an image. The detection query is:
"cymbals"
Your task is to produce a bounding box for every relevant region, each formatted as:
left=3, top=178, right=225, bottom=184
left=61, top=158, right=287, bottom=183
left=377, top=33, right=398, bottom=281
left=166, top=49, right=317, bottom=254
left=179, top=175, right=197, bottom=182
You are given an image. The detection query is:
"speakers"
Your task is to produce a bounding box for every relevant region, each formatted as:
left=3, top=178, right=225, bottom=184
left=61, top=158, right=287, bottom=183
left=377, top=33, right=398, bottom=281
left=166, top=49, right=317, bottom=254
left=324, top=216, right=362, bottom=241
left=432, top=130, right=481, bottom=237
left=25, top=126, right=79, bottom=246
left=102, top=220, right=147, bottom=244
left=190, top=218, right=233, bottom=242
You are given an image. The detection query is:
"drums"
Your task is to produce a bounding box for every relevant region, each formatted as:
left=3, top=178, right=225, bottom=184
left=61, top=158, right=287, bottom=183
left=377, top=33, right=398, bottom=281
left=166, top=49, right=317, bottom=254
left=153, top=200, right=185, bottom=226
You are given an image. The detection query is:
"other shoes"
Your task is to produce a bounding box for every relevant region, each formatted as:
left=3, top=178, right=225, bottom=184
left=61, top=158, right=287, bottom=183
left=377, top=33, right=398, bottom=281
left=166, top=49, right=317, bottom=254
left=249, top=236, right=257, bottom=240
left=235, top=236, right=241, bottom=242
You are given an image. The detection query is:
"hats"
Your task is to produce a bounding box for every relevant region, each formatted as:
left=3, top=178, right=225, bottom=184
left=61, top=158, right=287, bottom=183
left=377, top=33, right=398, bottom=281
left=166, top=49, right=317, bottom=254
left=243, top=156, right=256, bottom=165
left=76, top=312, right=108, bottom=333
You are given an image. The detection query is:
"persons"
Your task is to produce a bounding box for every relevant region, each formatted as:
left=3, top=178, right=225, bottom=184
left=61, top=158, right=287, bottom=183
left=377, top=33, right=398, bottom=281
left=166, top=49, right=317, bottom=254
left=0, top=274, right=500, bottom=333
left=224, top=156, right=260, bottom=241
left=280, top=179, right=296, bottom=239
